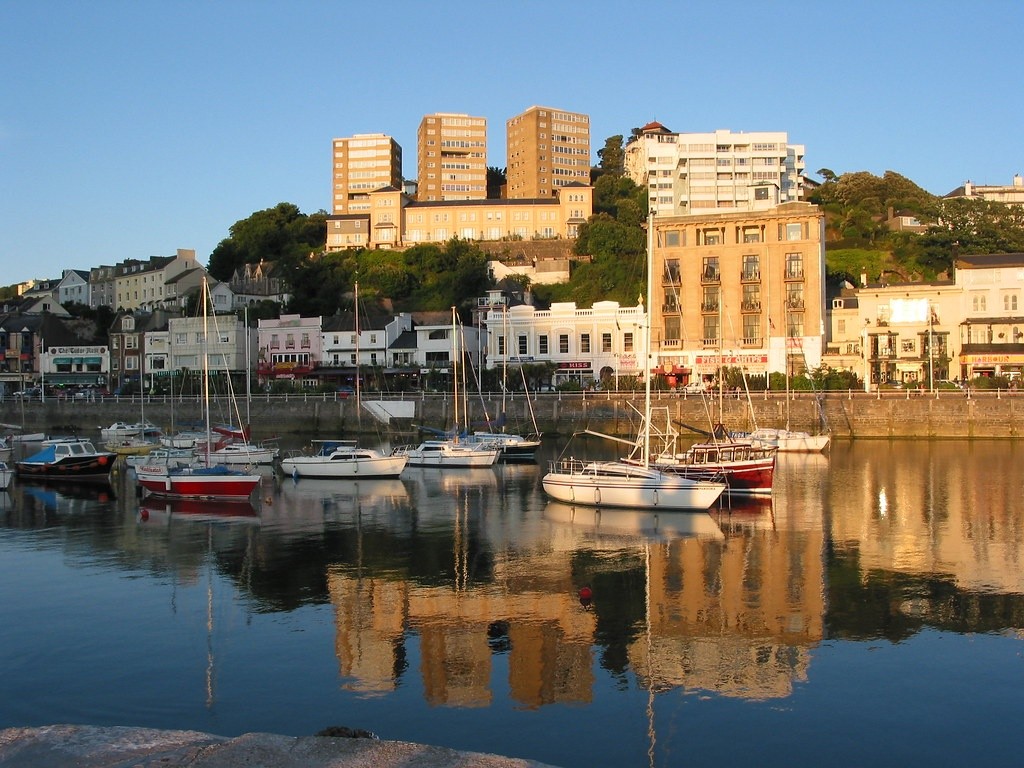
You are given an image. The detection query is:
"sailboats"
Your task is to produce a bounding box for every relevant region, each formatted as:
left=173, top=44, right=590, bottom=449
left=693, top=287, right=831, bottom=453
left=540, top=207, right=779, bottom=511
left=545, top=499, right=726, bottom=764
left=136, top=492, right=263, bottom=707
left=135, top=274, right=281, bottom=502
left=398, top=299, right=544, bottom=469
left=278, top=280, right=409, bottom=480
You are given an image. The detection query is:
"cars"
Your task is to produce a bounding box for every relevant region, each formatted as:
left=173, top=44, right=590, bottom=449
left=13, top=387, right=41, bottom=397
left=114, top=388, right=123, bottom=397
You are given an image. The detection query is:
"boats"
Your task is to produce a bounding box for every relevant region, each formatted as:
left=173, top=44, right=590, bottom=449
left=20, top=478, right=118, bottom=518
left=7, top=431, right=91, bottom=449
left=17, top=444, right=118, bottom=478
left=102, top=420, right=156, bottom=439
